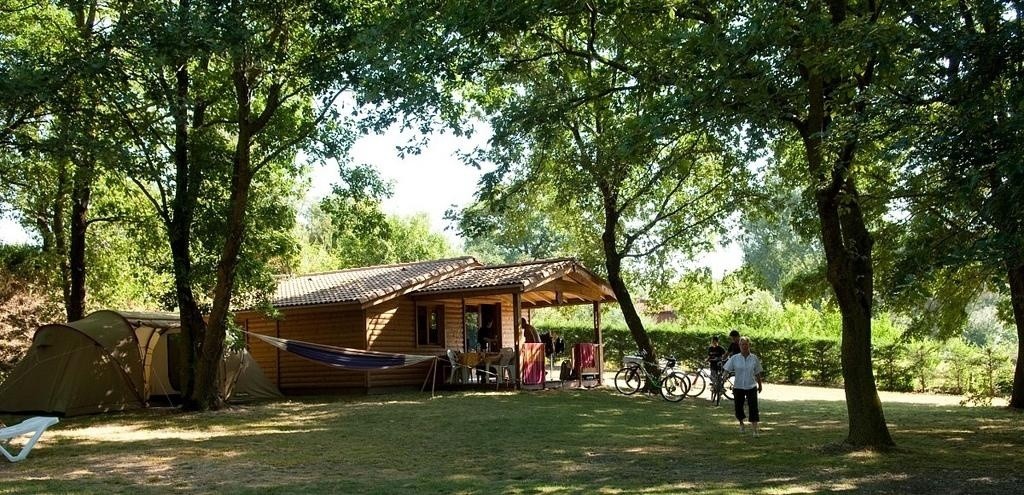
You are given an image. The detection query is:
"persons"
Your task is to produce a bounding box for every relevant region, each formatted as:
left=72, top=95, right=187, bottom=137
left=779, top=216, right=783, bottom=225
left=723, top=336, right=763, bottom=438
left=705, top=336, right=726, bottom=385
left=724, top=330, right=741, bottom=360
left=521, top=317, right=542, bottom=343
left=477, top=318, right=498, bottom=383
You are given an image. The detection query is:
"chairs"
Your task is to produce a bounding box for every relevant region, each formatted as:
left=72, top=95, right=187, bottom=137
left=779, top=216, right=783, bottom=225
left=443, top=347, right=516, bottom=391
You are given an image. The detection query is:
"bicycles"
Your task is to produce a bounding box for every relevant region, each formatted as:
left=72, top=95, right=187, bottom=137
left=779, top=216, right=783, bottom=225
left=680, top=355, right=747, bottom=406
left=614, top=344, right=691, bottom=402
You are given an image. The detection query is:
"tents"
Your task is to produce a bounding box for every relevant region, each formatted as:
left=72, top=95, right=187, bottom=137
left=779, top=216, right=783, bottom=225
left=0, top=310, right=284, bottom=418
